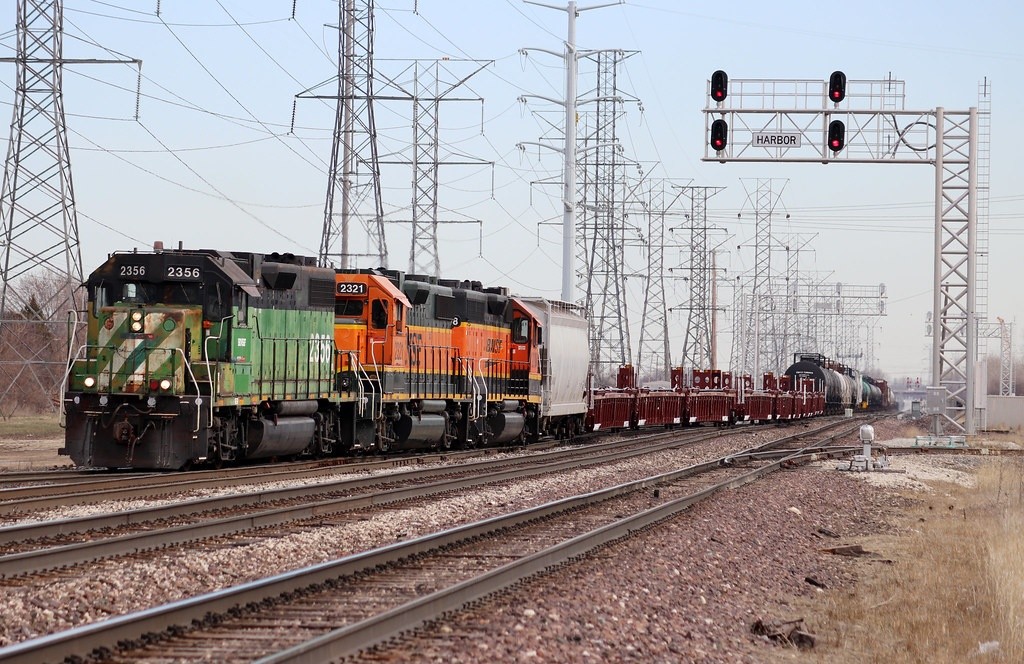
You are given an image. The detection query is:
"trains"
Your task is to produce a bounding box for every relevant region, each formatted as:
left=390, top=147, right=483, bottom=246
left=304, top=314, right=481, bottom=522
left=48, top=238, right=829, bottom=475
left=786, top=350, right=893, bottom=414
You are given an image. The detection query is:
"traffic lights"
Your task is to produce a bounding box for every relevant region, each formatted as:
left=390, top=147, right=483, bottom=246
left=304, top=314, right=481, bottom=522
left=828, top=71, right=847, bottom=103
left=710, top=70, right=729, bottom=102
left=827, top=120, right=845, bottom=151
left=710, top=119, right=728, bottom=151
